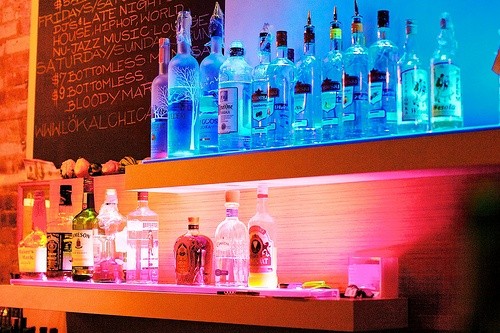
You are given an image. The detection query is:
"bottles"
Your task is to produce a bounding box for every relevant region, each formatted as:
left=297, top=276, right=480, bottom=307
left=167, top=11, right=199, bottom=159
left=250, top=30, right=295, bottom=150
left=431, top=12, right=463, bottom=131
left=150, top=38, right=171, bottom=159
left=396, top=18, right=429, bottom=134
left=198, top=2, right=227, bottom=155
left=126, top=191, right=159, bottom=285
left=46, top=184, right=75, bottom=280
left=212, top=190, right=248, bottom=287
left=367, top=9, right=399, bottom=139
left=343, top=1, right=367, bottom=141
left=321, top=5, right=343, bottom=142
left=294, top=9, right=321, bottom=146
left=248, top=183, right=278, bottom=288
left=218, top=40, right=252, bottom=153
left=72, top=177, right=98, bottom=282
left=17, top=189, right=47, bottom=281
left=172, top=216, right=213, bottom=286
left=91, top=188, right=126, bottom=283
left=0, top=315, right=58, bottom=333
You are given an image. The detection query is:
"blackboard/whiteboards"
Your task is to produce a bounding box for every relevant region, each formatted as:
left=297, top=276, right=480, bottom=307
left=26, top=0, right=225, bottom=169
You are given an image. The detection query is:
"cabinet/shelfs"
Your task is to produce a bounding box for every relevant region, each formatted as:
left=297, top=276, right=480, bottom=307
left=0, top=123, right=500, bottom=333
